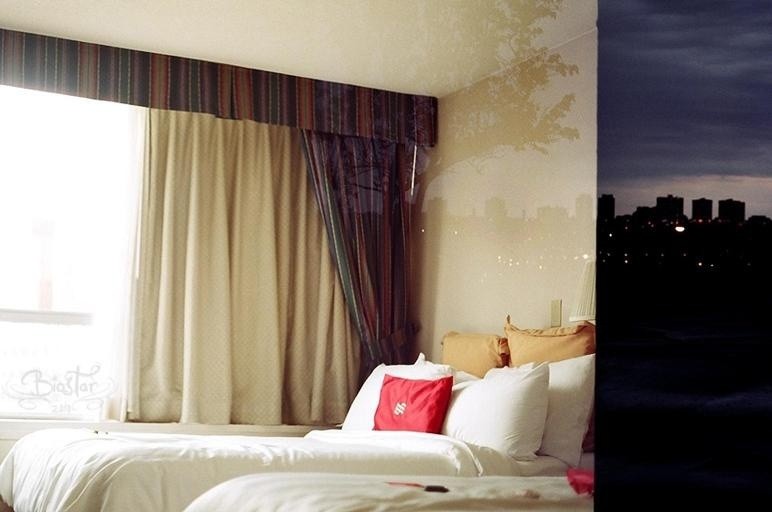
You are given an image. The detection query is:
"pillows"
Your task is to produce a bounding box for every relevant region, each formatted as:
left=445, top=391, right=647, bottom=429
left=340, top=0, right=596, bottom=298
left=504, top=316, right=597, bottom=368
left=372, top=373, right=453, bottom=435
left=337, top=362, right=451, bottom=431
left=440, top=361, right=552, bottom=460
left=440, top=331, right=509, bottom=379
left=537, top=352, right=598, bottom=468
left=414, top=351, right=483, bottom=384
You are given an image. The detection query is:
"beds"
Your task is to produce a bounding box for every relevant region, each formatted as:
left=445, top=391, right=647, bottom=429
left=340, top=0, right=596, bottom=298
left=184, top=473, right=595, bottom=512
left=2, top=425, right=595, bottom=511
